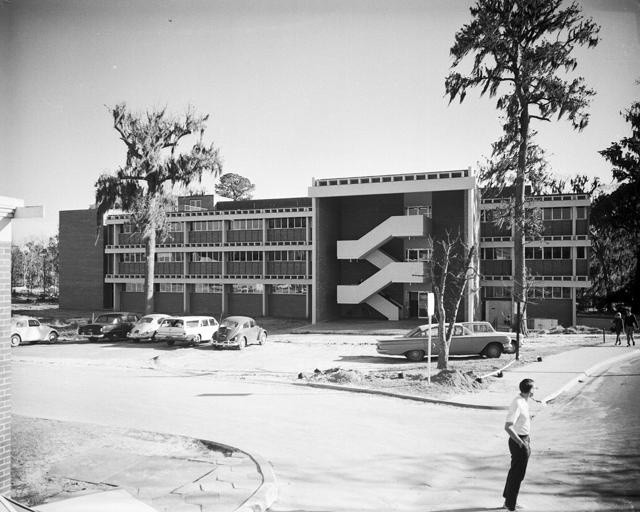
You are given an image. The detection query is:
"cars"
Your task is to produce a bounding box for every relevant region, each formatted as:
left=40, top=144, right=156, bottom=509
left=78, top=311, right=268, bottom=350
left=376, top=321, right=523, bottom=362
left=11, top=316, right=59, bottom=347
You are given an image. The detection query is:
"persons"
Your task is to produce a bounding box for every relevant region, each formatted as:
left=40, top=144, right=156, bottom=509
left=502, top=378, right=535, bottom=512
left=611, top=307, right=639, bottom=347
